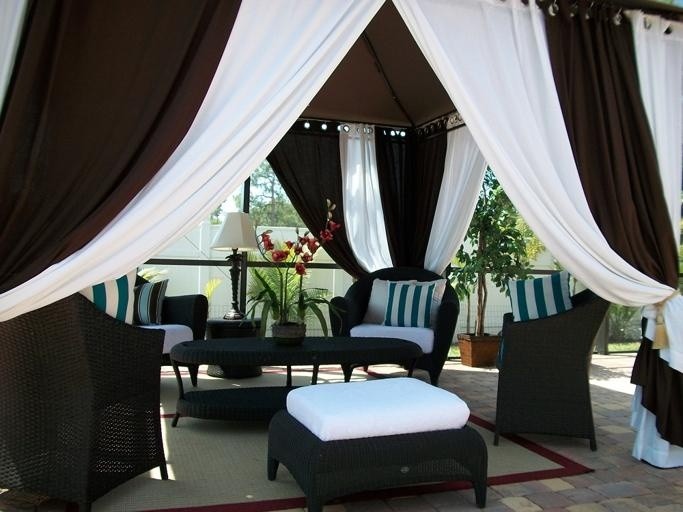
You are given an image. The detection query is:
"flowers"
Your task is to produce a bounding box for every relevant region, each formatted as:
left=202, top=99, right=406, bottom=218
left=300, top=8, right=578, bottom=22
left=239, top=199, right=346, bottom=339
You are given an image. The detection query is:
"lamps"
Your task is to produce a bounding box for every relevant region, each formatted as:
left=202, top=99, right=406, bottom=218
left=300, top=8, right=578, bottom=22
left=209, top=211, right=258, bottom=319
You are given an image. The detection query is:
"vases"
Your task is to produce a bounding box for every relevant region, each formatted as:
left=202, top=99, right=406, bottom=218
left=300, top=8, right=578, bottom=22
left=270, top=324, right=306, bottom=343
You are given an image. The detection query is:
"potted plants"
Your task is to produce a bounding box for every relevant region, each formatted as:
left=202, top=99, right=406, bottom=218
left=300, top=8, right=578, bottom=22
left=449, top=166, right=535, bottom=367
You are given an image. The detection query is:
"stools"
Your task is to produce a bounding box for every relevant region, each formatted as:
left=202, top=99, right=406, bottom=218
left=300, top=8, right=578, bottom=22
left=266, top=378, right=488, bottom=512
left=206, top=318, right=262, bottom=378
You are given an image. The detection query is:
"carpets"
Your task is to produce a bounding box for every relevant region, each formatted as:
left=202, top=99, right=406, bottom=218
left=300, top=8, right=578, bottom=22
left=0, top=366, right=596, bottom=512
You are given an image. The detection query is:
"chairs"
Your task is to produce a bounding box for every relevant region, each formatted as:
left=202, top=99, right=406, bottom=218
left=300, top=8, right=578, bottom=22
left=0, top=292, right=168, bottom=511
left=494, top=288, right=610, bottom=451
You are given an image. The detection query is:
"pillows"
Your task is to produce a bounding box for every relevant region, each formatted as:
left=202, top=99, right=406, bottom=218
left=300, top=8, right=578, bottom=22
left=366, top=278, right=418, bottom=326
left=395, top=278, right=447, bottom=327
left=135, top=279, right=168, bottom=325
left=380, top=281, right=436, bottom=328
left=80, top=268, right=137, bottom=324
left=508, top=269, right=573, bottom=321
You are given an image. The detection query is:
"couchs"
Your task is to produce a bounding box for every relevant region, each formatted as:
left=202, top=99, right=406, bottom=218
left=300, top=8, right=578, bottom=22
left=78, top=267, right=209, bottom=388
left=329, top=267, right=460, bottom=387
